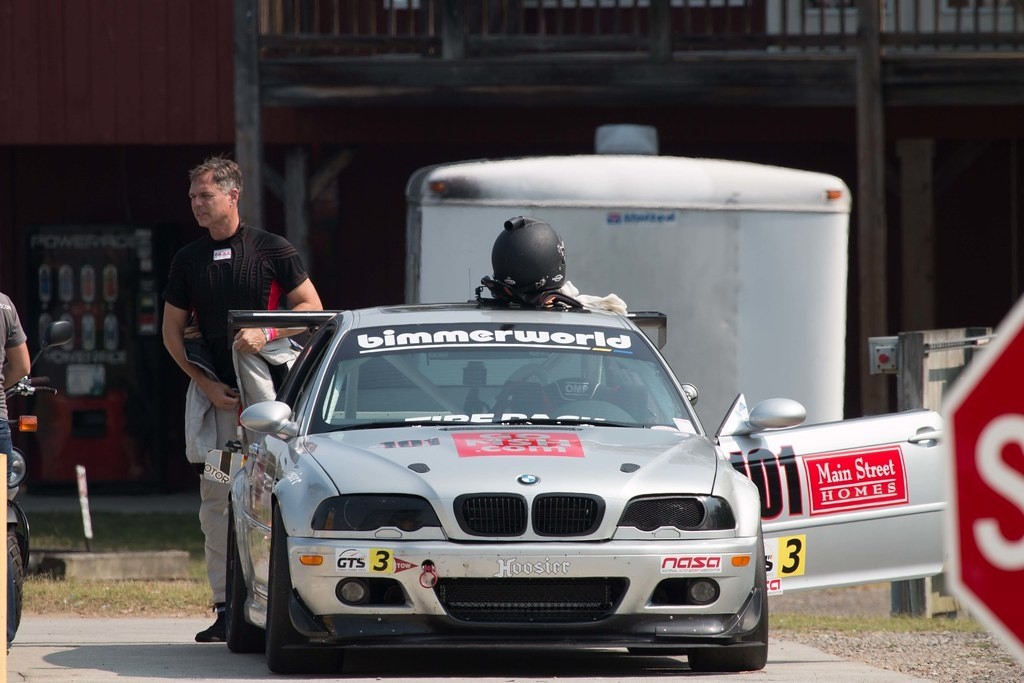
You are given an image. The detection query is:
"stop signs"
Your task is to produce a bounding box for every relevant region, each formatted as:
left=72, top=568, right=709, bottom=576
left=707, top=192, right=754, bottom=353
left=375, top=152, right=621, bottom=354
left=945, top=294, right=1024, bottom=666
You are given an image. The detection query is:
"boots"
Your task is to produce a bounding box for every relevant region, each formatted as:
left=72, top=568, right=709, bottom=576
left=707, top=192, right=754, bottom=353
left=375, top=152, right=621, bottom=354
left=195, top=602, right=226, bottom=642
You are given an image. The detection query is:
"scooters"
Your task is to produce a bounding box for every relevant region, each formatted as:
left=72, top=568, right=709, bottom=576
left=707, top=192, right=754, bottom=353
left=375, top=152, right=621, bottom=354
left=6, top=320, right=75, bottom=646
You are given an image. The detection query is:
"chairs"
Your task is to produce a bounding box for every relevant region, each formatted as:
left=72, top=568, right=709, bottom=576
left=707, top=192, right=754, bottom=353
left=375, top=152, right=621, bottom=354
left=546, top=378, right=607, bottom=408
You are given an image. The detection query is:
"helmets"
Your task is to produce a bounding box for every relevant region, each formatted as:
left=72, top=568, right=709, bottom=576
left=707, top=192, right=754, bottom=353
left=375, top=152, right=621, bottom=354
left=491, top=215, right=566, bottom=308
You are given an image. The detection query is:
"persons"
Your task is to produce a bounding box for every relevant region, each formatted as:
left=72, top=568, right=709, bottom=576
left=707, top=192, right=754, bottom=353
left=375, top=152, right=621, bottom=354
left=0, top=291, right=31, bottom=487
left=161, top=157, right=323, bottom=643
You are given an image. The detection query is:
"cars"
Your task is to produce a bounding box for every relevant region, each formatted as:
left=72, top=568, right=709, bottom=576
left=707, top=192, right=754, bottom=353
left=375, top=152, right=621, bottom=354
left=215, top=305, right=945, bottom=676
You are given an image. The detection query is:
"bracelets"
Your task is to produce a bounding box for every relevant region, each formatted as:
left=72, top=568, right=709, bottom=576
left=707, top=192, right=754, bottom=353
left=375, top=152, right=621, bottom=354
left=262, top=327, right=280, bottom=343
